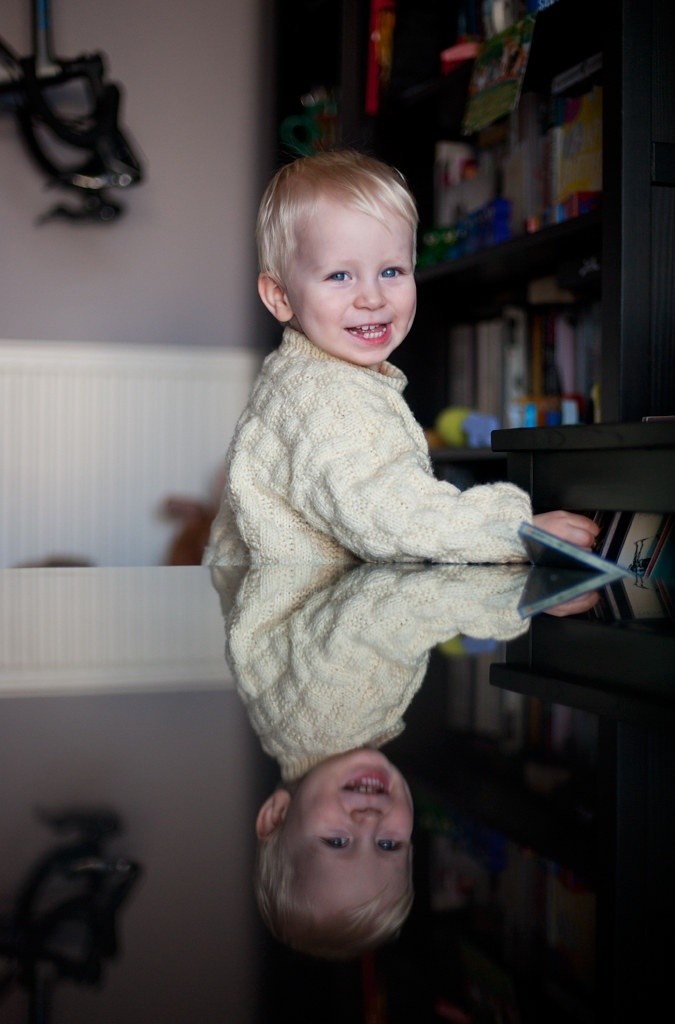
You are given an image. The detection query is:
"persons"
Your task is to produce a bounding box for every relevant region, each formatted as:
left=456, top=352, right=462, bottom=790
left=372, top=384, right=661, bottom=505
left=203, top=149, right=601, bottom=567
left=197, top=568, right=599, bottom=960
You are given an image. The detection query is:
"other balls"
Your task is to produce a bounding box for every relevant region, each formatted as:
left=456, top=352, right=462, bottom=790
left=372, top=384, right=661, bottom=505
left=436, top=406, right=474, bottom=450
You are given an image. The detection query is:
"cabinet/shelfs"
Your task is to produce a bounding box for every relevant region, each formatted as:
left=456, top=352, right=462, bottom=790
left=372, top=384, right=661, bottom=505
left=364, top=0, right=675, bottom=574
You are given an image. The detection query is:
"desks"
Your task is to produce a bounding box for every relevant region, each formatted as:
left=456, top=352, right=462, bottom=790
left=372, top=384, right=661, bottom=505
left=0, top=565, right=675, bottom=1024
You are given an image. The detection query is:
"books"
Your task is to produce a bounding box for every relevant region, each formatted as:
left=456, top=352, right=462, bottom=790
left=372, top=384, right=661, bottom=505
left=434, top=50, right=606, bottom=431
